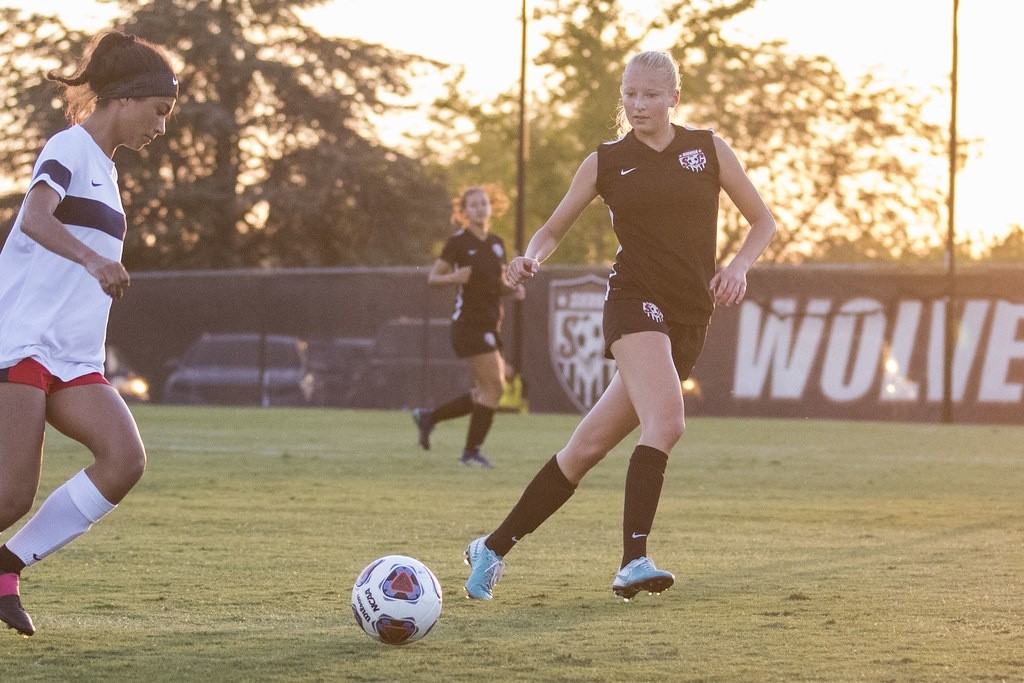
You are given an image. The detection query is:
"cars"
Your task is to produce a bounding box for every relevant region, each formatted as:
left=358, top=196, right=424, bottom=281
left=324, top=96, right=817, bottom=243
left=159, top=331, right=314, bottom=409
left=365, top=316, right=479, bottom=412
left=316, top=334, right=375, bottom=406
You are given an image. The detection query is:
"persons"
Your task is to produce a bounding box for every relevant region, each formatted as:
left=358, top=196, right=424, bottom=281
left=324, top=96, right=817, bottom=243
left=0, top=29, right=180, bottom=635
left=410, top=185, right=527, bottom=472
left=463, top=51, right=776, bottom=605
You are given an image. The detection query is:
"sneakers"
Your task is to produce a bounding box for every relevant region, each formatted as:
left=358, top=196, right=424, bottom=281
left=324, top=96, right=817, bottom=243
left=463, top=535, right=504, bottom=599
left=612, top=557, right=674, bottom=600
left=0, top=568, right=35, bottom=637
left=461, top=449, right=492, bottom=468
left=414, top=408, right=434, bottom=449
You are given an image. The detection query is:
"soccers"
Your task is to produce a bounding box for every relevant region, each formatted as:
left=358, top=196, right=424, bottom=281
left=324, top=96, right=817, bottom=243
left=350, top=556, right=442, bottom=646
